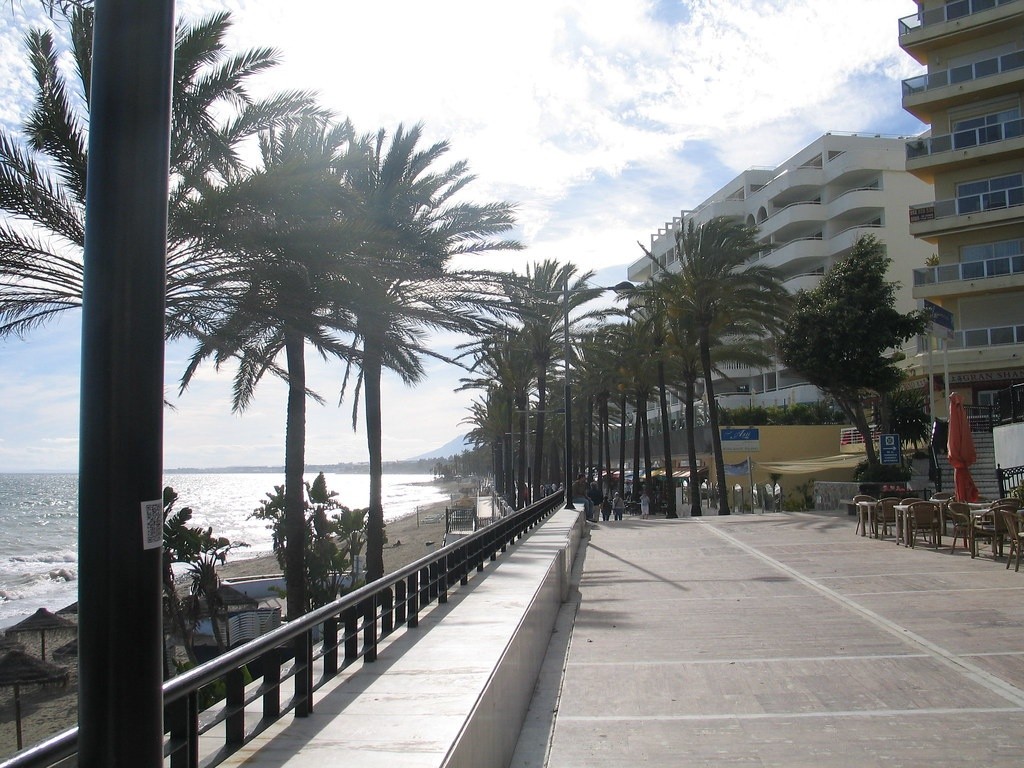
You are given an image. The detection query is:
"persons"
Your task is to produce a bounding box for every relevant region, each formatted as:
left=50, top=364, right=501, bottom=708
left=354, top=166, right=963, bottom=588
left=540, top=473, right=650, bottom=523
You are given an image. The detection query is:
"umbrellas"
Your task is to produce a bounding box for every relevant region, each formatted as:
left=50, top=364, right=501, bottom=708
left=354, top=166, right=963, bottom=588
left=0, top=650, right=70, bottom=751
left=5, top=608, right=78, bottom=664
left=947, top=392, right=979, bottom=548
left=52, top=638, right=78, bottom=659
left=55, top=601, right=78, bottom=614
left=189, top=584, right=258, bottom=647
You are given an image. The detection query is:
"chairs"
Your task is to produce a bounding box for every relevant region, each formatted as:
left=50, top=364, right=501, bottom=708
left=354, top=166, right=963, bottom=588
left=852, top=492, right=1024, bottom=573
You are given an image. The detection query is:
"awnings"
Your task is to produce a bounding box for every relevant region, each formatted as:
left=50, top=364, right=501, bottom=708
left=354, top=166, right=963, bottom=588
left=603, top=467, right=707, bottom=479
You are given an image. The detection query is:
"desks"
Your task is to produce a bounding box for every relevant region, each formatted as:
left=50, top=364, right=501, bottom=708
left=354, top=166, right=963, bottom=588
left=967, top=509, right=1024, bottom=557
left=930, top=499, right=952, bottom=535
left=859, top=502, right=882, bottom=539
left=968, top=503, right=994, bottom=522
left=893, top=505, right=913, bottom=547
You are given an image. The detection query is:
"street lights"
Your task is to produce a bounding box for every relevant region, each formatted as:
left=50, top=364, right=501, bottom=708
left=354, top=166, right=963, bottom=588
left=564, top=274, right=637, bottom=509
left=525, top=392, right=565, bottom=507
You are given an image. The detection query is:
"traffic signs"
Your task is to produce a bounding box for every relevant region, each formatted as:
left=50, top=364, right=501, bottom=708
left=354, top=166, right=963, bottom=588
left=879, top=434, right=901, bottom=466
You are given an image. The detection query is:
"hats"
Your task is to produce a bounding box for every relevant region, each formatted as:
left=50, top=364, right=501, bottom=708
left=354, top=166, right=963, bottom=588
left=615, top=492, right=619, bottom=495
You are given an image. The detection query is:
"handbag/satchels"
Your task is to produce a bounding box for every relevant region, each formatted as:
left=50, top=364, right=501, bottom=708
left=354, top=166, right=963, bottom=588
left=611, top=509, right=615, bottom=515
